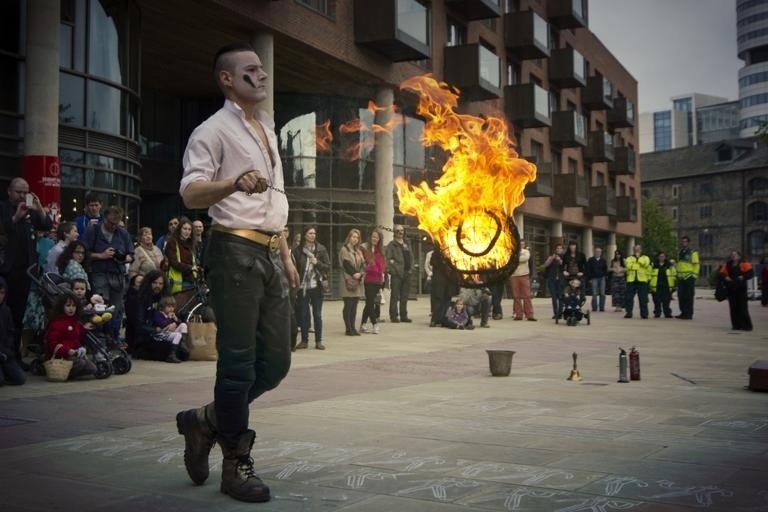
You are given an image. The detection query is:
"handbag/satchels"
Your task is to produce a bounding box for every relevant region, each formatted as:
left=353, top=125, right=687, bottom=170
left=714, top=279, right=728, bottom=302
left=317, top=279, right=332, bottom=297
left=43, top=343, right=73, bottom=383
left=187, top=315, right=219, bottom=361
left=344, top=277, right=359, bottom=293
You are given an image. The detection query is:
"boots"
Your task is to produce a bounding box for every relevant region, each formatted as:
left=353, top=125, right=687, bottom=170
left=174, top=402, right=221, bottom=486
left=217, top=429, right=273, bottom=504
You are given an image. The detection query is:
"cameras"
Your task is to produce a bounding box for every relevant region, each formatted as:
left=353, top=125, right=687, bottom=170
left=115, top=249, right=126, bottom=261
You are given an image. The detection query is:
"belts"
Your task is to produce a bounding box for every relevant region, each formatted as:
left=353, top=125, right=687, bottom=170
left=209, top=222, right=284, bottom=254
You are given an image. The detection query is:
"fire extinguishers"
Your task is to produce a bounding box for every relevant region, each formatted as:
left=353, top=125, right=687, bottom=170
left=628, top=346, right=640, bottom=380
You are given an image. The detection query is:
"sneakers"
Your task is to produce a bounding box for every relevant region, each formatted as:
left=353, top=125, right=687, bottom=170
left=360, top=324, right=381, bottom=334
left=296, top=339, right=309, bottom=349
left=513, top=316, right=540, bottom=322
left=623, top=313, right=693, bottom=320
left=315, top=341, right=326, bottom=349
left=345, top=329, right=361, bottom=336
left=392, top=316, right=413, bottom=324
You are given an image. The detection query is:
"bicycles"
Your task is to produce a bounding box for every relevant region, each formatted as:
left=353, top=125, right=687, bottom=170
left=177, top=261, right=215, bottom=325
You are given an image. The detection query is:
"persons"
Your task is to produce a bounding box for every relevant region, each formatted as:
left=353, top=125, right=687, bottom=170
left=544, top=244, right=566, bottom=319
left=340, top=226, right=414, bottom=337
left=563, top=277, right=582, bottom=309
left=561, top=240, right=586, bottom=277
left=425, top=239, right=537, bottom=329
left=0, top=177, right=216, bottom=385
left=719, top=250, right=754, bottom=330
left=756, top=256, right=768, bottom=306
left=175, top=40, right=303, bottom=503
left=675, top=236, right=700, bottom=320
left=622, top=245, right=652, bottom=319
left=607, top=250, right=627, bottom=312
left=649, top=252, right=677, bottom=317
left=277, top=225, right=330, bottom=350
left=585, top=247, right=608, bottom=312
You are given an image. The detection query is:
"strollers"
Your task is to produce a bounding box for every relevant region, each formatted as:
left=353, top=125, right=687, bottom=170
left=556, top=269, right=591, bottom=327
left=26, top=262, right=132, bottom=382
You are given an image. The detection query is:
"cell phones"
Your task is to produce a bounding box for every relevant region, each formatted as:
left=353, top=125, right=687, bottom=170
left=25, top=193, right=33, bottom=207
